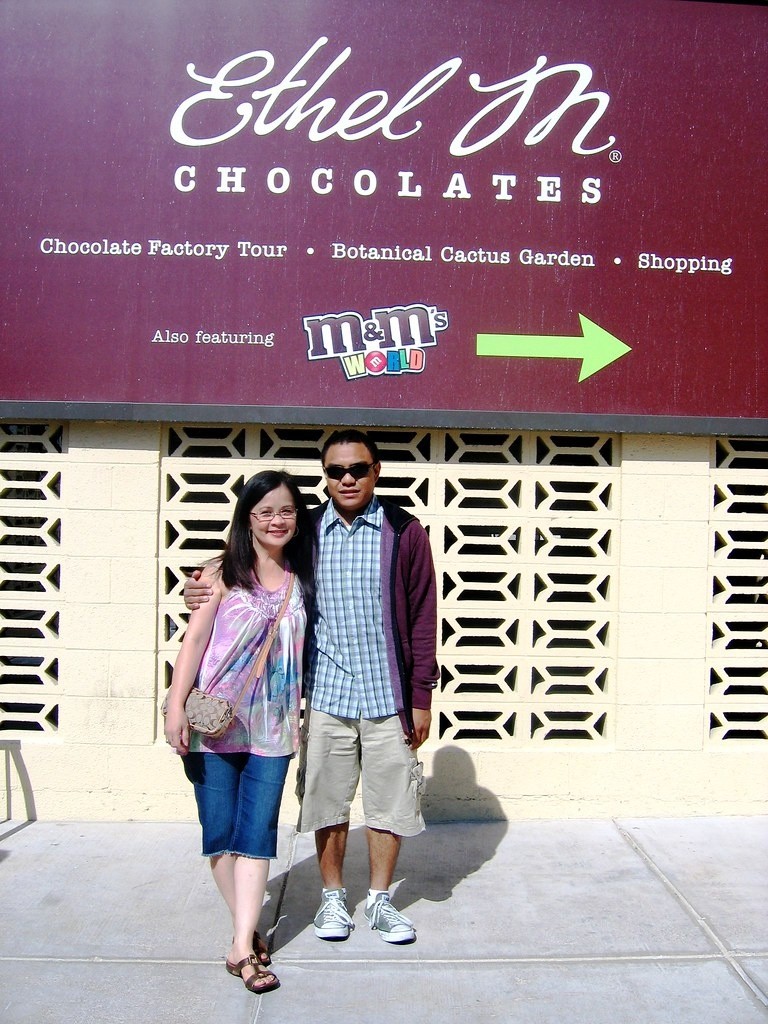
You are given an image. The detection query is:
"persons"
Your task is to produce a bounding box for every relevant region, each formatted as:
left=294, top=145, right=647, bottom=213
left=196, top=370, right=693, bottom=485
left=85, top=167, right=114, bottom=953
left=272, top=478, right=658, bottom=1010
left=184, top=428, right=440, bottom=943
left=164, top=469, right=310, bottom=992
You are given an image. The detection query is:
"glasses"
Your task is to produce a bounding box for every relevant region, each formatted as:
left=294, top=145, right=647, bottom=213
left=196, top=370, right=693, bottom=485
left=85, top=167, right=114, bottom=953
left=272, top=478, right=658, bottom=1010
left=250, top=506, right=295, bottom=522
left=322, top=462, right=374, bottom=480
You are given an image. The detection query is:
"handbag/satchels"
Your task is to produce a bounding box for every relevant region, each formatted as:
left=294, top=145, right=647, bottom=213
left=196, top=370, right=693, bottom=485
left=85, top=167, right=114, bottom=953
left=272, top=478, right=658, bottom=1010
left=161, top=686, right=232, bottom=739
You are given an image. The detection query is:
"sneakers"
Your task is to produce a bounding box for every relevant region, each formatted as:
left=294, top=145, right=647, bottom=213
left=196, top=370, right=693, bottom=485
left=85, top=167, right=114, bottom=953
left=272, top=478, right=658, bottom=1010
left=314, top=887, right=354, bottom=940
left=364, top=889, right=416, bottom=943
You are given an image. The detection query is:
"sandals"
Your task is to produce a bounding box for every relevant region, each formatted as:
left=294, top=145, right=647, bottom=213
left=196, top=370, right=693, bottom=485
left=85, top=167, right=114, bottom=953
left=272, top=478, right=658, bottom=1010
left=253, top=930, right=271, bottom=965
left=224, top=954, right=281, bottom=993
